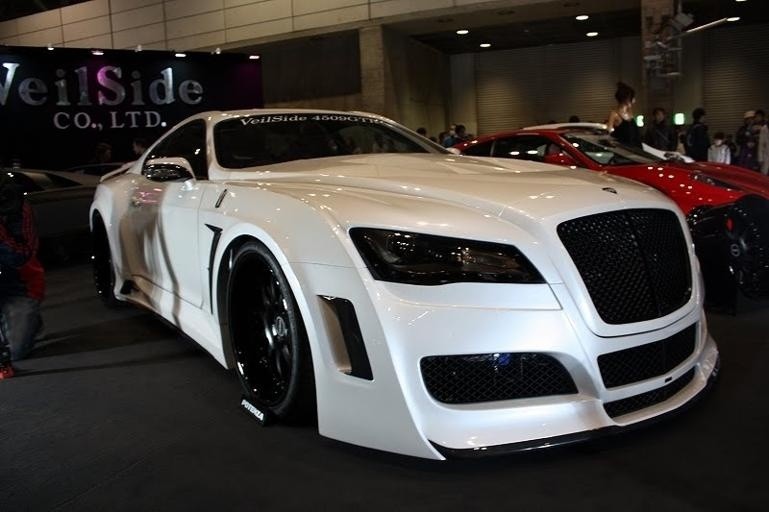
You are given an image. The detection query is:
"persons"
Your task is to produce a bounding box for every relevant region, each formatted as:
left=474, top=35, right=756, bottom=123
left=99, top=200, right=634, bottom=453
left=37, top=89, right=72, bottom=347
left=128, top=138, right=146, bottom=155
left=567, top=114, right=581, bottom=122
left=609, top=79, right=643, bottom=154
left=0, top=173, right=47, bottom=379
left=650, top=107, right=769, bottom=174
left=345, top=123, right=472, bottom=154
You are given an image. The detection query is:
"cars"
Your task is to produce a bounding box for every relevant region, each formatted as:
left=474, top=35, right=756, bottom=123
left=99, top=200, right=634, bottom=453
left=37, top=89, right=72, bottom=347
left=0, top=162, right=130, bottom=268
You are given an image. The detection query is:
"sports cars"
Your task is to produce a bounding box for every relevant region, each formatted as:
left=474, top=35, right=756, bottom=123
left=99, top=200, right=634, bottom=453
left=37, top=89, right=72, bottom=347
left=447, top=119, right=768, bottom=315
